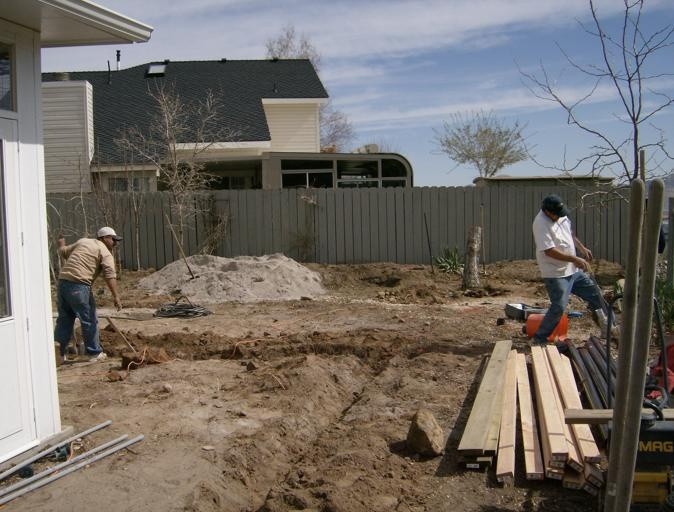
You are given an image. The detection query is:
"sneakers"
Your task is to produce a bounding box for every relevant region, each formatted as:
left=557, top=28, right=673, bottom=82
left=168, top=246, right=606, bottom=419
left=60, top=356, right=66, bottom=364
left=88, top=352, right=107, bottom=362
left=528, top=337, right=549, bottom=348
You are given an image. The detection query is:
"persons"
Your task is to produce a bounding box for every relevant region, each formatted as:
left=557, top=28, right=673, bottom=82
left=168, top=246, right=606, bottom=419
left=53, top=226, right=125, bottom=368
left=530, top=195, right=616, bottom=342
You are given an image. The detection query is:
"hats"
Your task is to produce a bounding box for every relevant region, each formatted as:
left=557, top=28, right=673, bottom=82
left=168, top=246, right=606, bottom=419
left=98, top=227, right=123, bottom=241
left=542, top=194, right=569, bottom=216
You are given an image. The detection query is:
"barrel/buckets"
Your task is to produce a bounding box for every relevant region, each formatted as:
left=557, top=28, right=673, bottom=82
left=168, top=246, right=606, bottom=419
left=526, top=309, right=568, bottom=340
